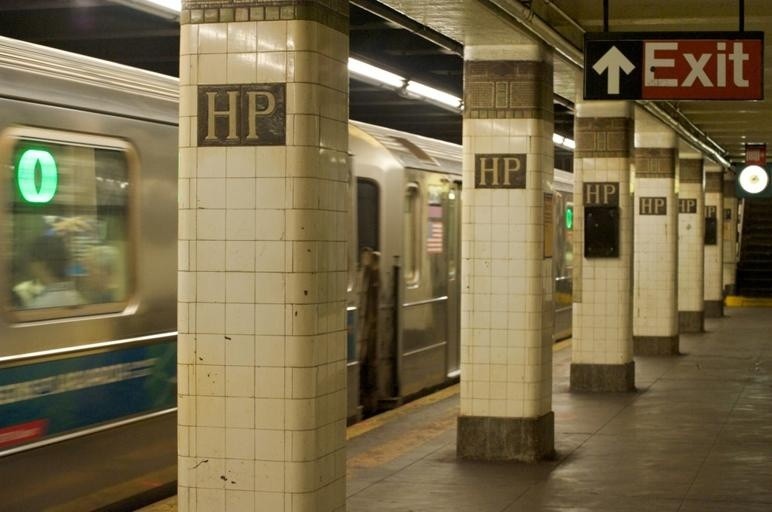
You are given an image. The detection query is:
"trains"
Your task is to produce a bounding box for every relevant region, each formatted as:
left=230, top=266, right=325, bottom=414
left=0, top=34, right=575, bottom=511
